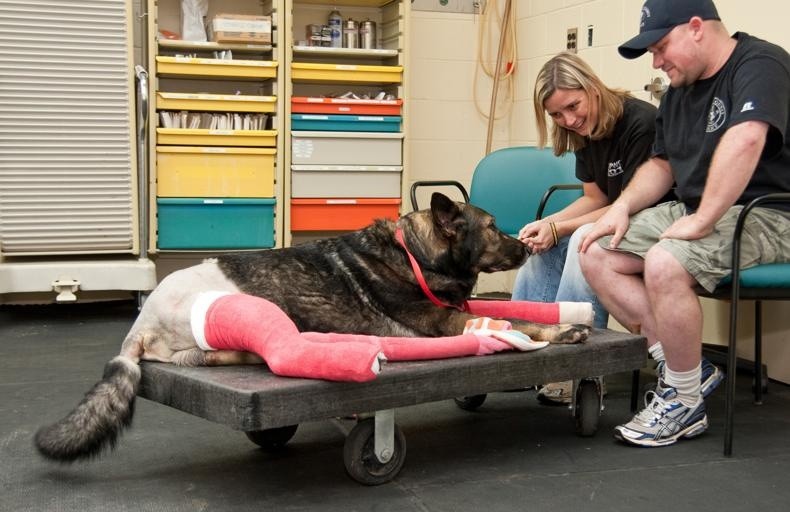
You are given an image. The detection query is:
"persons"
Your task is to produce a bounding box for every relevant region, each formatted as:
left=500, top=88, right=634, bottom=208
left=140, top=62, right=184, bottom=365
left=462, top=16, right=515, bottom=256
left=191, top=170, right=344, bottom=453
left=511, top=51, right=677, bottom=407
left=577, top=0, right=790, bottom=448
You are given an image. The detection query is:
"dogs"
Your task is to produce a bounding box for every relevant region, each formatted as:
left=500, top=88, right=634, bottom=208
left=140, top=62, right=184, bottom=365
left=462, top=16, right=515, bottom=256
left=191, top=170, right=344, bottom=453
left=34, top=192, right=596, bottom=466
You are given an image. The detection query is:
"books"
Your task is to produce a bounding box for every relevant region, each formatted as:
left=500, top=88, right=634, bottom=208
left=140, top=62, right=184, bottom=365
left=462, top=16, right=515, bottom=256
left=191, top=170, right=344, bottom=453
left=175, top=50, right=232, bottom=60
left=160, top=110, right=268, bottom=130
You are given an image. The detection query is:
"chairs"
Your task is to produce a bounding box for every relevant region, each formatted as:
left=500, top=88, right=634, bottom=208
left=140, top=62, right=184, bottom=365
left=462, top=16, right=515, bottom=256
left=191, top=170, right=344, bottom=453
left=409, top=146, right=583, bottom=238
left=631, top=192, right=789, bottom=454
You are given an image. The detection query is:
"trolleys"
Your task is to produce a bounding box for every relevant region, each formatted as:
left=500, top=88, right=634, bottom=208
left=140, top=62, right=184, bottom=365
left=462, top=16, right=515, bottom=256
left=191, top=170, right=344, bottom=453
left=136, top=326, right=647, bottom=487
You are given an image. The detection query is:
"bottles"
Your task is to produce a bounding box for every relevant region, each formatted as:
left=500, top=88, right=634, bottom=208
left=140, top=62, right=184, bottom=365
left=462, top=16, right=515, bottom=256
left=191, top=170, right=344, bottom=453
left=342, top=15, right=359, bottom=50
left=359, top=16, right=377, bottom=51
left=327, top=6, right=343, bottom=49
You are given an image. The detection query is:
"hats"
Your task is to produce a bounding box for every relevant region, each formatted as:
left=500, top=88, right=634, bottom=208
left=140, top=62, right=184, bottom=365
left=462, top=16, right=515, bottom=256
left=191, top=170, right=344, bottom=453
left=619, top=1, right=721, bottom=59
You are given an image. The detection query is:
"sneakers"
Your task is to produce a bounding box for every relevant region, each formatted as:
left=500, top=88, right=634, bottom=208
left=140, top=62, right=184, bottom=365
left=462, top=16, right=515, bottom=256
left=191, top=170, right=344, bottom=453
left=536, top=379, right=608, bottom=403
left=614, top=355, right=726, bottom=447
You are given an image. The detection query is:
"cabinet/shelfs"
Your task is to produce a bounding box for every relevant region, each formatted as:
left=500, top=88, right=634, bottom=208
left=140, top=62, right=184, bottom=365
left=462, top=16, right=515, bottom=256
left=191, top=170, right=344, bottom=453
left=140, top=0, right=410, bottom=260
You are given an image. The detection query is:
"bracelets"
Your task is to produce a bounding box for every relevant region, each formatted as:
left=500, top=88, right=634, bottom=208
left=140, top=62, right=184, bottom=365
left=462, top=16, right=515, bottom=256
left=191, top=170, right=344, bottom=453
left=549, top=222, right=559, bottom=247
